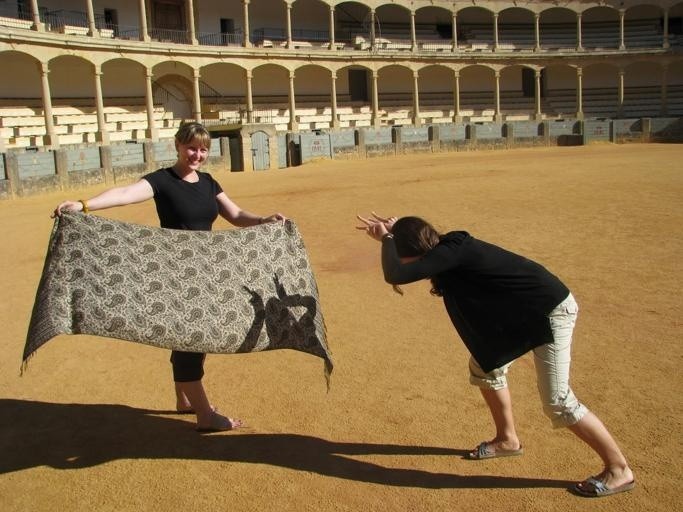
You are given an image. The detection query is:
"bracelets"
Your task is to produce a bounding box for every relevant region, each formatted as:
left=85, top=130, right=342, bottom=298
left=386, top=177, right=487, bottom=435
left=78, top=200, right=88, bottom=214
left=381, top=232, right=393, bottom=240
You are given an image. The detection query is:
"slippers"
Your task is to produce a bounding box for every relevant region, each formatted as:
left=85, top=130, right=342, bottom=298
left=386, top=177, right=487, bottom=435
left=177, top=405, right=243, bottom=432
left=466, top=441, right=523, bottom=460
left=573, top=475, right=635, bottom=497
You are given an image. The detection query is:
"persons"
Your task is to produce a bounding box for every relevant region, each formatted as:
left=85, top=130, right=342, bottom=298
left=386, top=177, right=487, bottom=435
left=353, top=209, right=637, bottom=499
left=50, top=120, right=291, bottom=432
